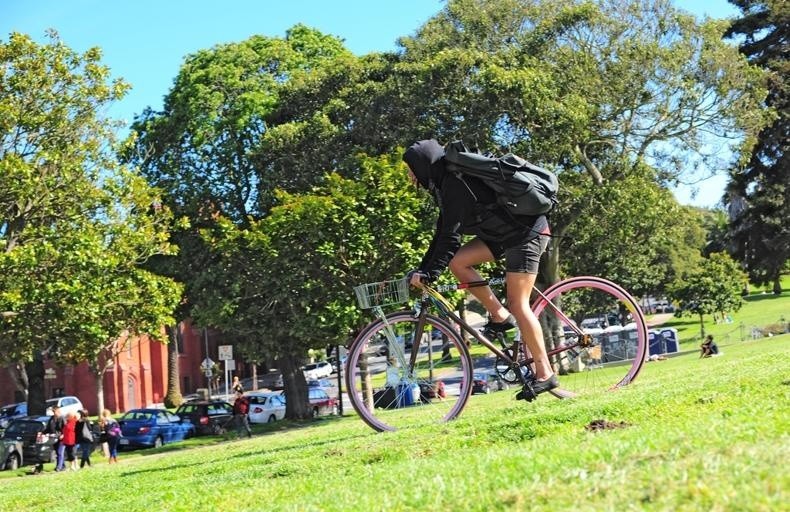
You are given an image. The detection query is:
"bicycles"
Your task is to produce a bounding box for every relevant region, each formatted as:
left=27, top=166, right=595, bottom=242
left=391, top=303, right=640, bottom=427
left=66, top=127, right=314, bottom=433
left=346, top=271, right=650, bottom=432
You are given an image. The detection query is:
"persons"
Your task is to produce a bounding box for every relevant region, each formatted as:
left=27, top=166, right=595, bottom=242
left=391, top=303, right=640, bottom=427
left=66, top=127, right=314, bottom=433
left=41, top=405, right=122, bottom=471
left=386, top=356, right=400, bottom=388
left=646, top=354, right=668, bottom=363
left=232, top=390, right=252, bottom=440
left=698, top=334, right=719, bottom=358
left=233, top=376, right=243, bottom=396
left=713, top=310, right=734, bottom=325
left=403, top=139, right=559, bottom=401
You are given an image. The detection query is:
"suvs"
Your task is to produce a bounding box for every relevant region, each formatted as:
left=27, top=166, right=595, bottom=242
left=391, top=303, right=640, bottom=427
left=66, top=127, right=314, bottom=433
left=46, top=397, right=83, bottom=421
left=5, top=415, right=60, bottom=463
left=280, top=387, right=338, bottom=422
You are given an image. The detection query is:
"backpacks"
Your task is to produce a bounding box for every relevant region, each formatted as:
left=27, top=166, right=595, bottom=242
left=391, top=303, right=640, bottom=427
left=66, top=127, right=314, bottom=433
left=441, top=139, right=561, bottom=218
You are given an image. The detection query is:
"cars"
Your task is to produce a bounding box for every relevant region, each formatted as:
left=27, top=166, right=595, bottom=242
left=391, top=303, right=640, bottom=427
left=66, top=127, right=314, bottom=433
left=273, top=374, right=284, bottom=389
left=0, top=402, right=29, bottom=430
left=0, top=434, right=24, bottom=470
left=240, top=391, right=287, bottom=424
left=362, top=379, right=446, bottom=409
left=327, top=327, right=447, bottom=377
left=175, top=398, right=235, bottom=437
left=116, top=409, right=197, bottom=450
left=303, top=360, right=333, bottom=380
left=460, top=371, right=511, bottom=394
left=561, top=297, right=675, bottom=339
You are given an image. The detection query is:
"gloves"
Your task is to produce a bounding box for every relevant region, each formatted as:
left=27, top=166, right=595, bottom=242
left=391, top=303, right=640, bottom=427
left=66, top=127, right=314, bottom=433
left=405, top=269, right=435, bottom=290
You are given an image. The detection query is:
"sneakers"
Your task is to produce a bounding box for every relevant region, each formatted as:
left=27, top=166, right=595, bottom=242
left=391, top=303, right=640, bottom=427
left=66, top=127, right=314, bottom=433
left=515, top=374, right=562, bottom=402
left=54, top=454, right=122, bottom=474
left=477, top=311, right=518, bottom=347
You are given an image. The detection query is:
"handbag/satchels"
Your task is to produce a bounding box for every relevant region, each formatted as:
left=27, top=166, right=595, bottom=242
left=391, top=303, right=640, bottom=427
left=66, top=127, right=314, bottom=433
left=81, top=422, right=94, bottom=443
left=99, top=430, right=107, bottom=444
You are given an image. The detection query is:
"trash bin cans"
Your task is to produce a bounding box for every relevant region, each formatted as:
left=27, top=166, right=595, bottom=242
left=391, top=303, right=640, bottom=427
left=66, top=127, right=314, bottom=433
left=562, top=314, right=680, bottom=366
